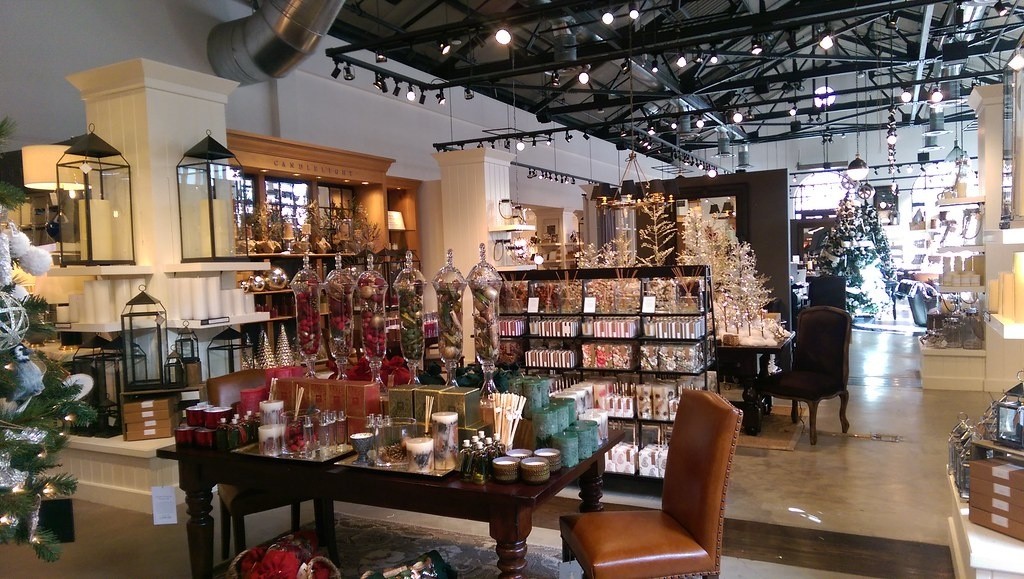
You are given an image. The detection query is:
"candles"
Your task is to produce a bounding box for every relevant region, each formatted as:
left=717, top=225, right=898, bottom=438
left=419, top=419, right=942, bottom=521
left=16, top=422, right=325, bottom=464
left=808, top=259, right=812, bottom=270
left=201, top=198, right=230, bottom=257
left=957, top=184, right=967, bottom=198
left=79, top=199, right=112, bottom=260
left=945, top=192, right=952, bottom=199
left=988, top=252, right=1023, bottom=322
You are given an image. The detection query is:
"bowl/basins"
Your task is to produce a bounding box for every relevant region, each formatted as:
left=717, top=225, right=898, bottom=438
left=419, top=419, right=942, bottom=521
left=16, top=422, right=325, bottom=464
left=505, top=448, right=532, bottom=460
left=491, top=456, right=520, bottom=483
left=533, top=447, right=561, bottom=472
left=520, top=457, right=550, bottom=485
left=174, top=404, right=233, bottom=448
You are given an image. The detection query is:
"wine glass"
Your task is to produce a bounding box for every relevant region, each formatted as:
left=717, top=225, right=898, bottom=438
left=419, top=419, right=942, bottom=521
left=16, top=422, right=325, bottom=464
left=350, top=432, right=374, bottom=466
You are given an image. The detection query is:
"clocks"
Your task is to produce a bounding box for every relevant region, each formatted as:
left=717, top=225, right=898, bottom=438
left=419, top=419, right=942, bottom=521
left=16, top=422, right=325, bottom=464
left=56, top=275, right=255, bottom=323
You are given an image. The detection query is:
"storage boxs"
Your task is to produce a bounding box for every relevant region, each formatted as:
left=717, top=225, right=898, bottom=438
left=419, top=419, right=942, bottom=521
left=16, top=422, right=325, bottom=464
left=723, top=334, right=739, bottom=345
left=969, top=457, right=1024, bottom=542
left=123, top=397, right=181, bottom=441
left=275, top=376, right=489, bottom=446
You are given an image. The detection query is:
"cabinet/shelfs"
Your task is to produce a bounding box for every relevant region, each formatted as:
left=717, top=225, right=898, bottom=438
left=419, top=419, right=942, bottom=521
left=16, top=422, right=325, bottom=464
left=946, top=435, right=1023, bottom=579
left=488, top=223, right=538, bottom=272
left=235, top=253, right=356, bottom=325
left=497, top=265, right=708, bottom=494
left=48, top=262, right=270, bottom=332
left=918, top=197, right=986, bottom=392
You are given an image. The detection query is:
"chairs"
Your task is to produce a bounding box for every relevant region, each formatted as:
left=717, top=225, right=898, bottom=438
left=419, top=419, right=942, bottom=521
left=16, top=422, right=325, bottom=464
left=207, top=366, right=342, bottom=568
left=559, top=387, right=743, bottom=579
left=759, top=306, right=852, bottom=445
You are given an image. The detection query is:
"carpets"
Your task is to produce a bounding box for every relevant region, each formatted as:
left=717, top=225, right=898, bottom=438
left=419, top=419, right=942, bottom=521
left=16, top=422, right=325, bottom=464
left=730, top=401, right=807, bottom=451
left=212, top=512, right=562, bottom=578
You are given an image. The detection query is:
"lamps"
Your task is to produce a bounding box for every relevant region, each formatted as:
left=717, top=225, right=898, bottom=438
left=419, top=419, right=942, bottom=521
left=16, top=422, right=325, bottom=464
left=388, top=211, right=406, bottom=245
left=22, top=145, right=92, bottom=241
left=651, top=58, right=659, bottom=72
left=326, top=0, right=943, bottom=184
left=590, top=0, right=680, bottom=217
left=790, top=107, right=798, bottom=115
left=828, top=135, right=834, bottom=143
left=821, top=135, right=827, bottom=145
left=943, top=81, right=972, bottom=170
left=846, top=15, right=869, bottom=181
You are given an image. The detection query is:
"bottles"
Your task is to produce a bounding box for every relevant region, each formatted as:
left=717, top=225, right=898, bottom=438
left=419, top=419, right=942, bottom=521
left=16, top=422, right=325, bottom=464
left=459, top=440, right=472, bottom=482
left=485, top=436, right=496, bottom=476
left=471, top=435, right=478, bottom=450
left=478, top=431, right=485, bottom=442
left=472, top=441, right=487, bottom=484
left=493, top=433, right=505, bottom=456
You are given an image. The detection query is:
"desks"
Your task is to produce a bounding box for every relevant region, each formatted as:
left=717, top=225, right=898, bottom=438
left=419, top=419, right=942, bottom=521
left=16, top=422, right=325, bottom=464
left=156, top=429, right=626, bottom=579
left=717, top=331, right=795, bottom=436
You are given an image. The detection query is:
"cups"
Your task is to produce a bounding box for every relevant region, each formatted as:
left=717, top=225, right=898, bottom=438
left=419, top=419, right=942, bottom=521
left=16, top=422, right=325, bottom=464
left=377, top=417, right=417, bottom=467
left=513, top=375, right=609, bottom=467
left=279, top=409, right=322, bottom=455
